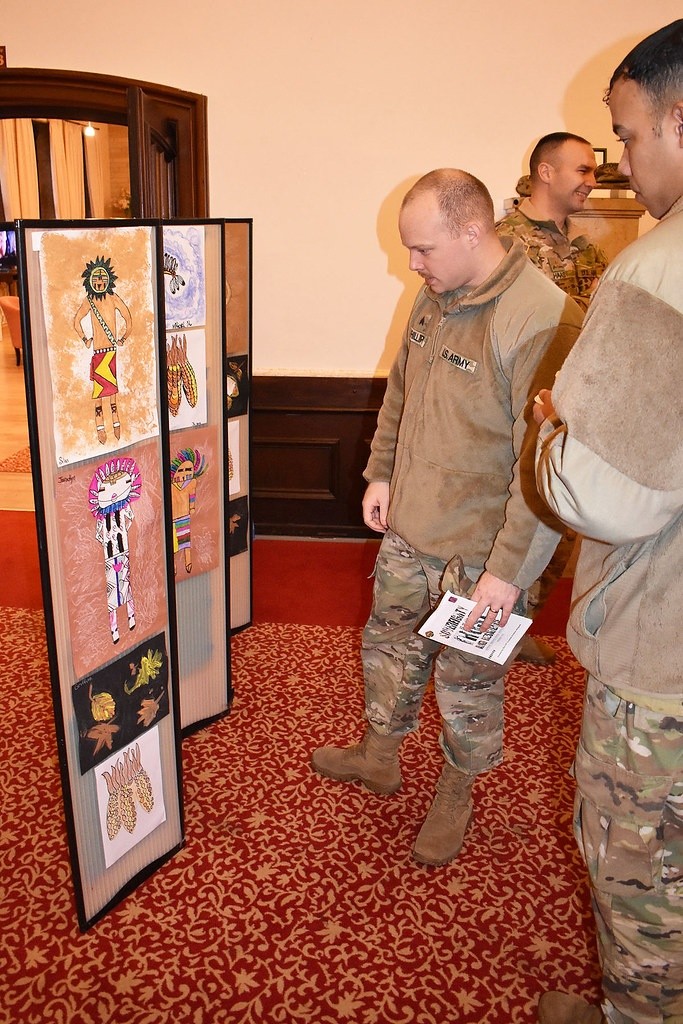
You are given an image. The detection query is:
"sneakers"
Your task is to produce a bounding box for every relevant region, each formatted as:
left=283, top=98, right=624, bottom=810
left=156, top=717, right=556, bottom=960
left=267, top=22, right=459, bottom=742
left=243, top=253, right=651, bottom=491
left=539, top=990, right=608, bottom=1024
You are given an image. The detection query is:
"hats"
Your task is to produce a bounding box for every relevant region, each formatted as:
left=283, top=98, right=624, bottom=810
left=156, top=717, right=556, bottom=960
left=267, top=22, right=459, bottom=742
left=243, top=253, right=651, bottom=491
left=595, top=163, right=630, bottom=182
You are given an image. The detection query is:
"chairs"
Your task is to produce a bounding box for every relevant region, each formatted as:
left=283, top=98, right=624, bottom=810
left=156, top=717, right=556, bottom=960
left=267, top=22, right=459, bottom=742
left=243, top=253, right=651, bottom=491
left=0, top=296, right=23, bottom=366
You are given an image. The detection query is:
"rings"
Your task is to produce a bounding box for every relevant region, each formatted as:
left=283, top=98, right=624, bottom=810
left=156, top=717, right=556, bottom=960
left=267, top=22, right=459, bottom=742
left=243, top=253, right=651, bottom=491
left=489, top=608, right=498, bottom=613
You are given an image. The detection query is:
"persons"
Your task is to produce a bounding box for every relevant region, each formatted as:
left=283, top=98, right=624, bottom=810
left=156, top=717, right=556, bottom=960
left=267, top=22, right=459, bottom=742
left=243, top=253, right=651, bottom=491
left=311, top=169, right=585, bottom=868
left=492, top=132, right=610, bottom=663
left=530, top=18, right=682, bottom=1024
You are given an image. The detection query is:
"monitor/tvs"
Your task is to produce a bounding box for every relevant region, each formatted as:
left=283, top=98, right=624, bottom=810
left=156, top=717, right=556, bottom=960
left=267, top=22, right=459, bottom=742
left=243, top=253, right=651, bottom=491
left=0, top=222, right=18, bottom=272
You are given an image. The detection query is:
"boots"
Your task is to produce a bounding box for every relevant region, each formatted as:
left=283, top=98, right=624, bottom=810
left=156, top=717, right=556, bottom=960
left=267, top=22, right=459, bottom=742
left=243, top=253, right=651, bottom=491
left=412, top=763, right=475, bottom=867
left=309, top=717, right=408, bottom=794
